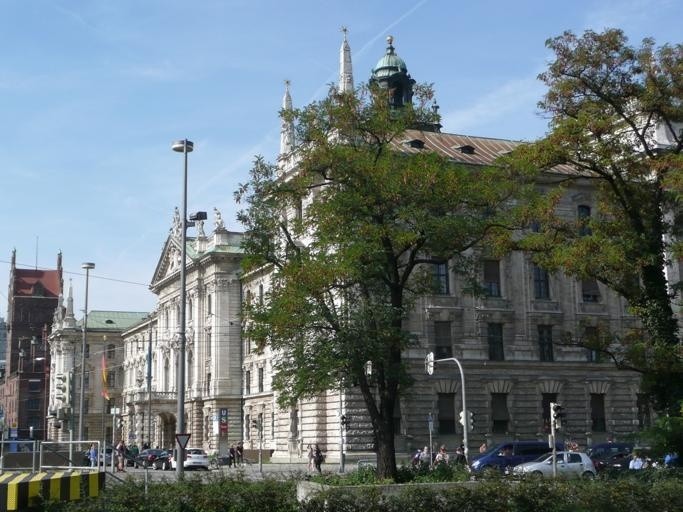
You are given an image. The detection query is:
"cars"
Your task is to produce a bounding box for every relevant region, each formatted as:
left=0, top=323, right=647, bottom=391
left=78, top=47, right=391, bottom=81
left=584, top=441, right=634, bottom=471
left=133, top=449, right=169, bottom=470
left=169, top=448, right=211, bottom=471
left=512, top=451, right=596, bottom=484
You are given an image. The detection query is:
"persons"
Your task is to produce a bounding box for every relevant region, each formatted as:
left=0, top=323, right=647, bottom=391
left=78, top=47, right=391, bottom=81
left=307, top=444, right=324, bottom=474
left=79, top=443, right=98, bottom=467
left=566, top=439, right=579, bottom=452
left=629, top=448, right=678, bottom=470
left=229, top=441, right=243, bottom=467
left=115, top=438, right=171, bottom=472
left=412, top=440, right=488, bottom=472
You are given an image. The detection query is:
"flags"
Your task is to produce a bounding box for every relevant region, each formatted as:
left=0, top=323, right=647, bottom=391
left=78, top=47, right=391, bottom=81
left=100, top=347, right=111, bottom=401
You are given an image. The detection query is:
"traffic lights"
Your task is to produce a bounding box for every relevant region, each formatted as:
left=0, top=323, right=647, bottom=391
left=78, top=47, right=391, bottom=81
left=553, top=404, right=561, bottom=430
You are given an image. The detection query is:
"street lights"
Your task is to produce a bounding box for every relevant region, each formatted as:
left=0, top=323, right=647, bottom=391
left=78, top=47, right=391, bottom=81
left=171, top=138, right=194, bottom=480
left=77, top=262, right=96, bottom=452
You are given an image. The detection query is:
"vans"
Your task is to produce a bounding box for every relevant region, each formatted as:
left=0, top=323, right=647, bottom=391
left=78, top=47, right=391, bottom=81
left=470, top=439, right=565, bottom=481
